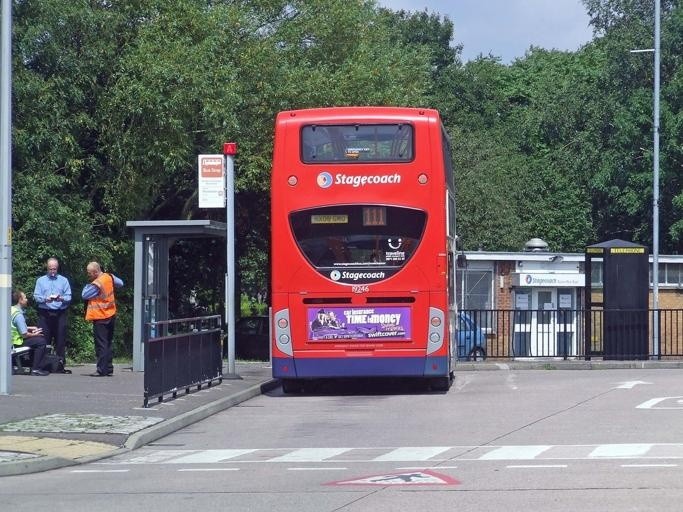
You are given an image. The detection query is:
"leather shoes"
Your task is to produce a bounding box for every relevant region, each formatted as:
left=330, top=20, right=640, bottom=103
left=32, top=369, right=49, bottom=376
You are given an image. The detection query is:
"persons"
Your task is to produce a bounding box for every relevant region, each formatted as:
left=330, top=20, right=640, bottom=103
left=9, top=291, right=50, bottom=376
left=32, top=258, right=73, bottom=374
left=81, top=262, right=123, bottom=377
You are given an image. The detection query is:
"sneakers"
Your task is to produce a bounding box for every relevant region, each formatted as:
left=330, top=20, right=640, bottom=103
left=91, top=370, right=113, bottom=376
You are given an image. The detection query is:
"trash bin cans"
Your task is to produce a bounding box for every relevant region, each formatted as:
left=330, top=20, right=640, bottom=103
left=236, top=316, right=270, bottom=361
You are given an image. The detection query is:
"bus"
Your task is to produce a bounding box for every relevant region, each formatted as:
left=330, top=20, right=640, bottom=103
left=268, top=105, right=455, bottom=390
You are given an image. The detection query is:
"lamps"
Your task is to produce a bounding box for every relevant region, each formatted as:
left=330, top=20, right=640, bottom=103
left=499, top=270, right=505, bottom=289
left=518, top=260, right=523, bottom=267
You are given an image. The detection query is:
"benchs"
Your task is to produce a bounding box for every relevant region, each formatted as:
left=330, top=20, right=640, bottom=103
left=11, top=344, right=54, bottom=374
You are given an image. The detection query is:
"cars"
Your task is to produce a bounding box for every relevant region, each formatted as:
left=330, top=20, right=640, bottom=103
left=223, top=316, right=270, bottom=362
left=456, top=310, right=488, bottom=361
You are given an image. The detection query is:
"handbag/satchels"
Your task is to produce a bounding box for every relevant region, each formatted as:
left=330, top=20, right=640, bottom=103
left=40, top=354, right=71, bottom=374
